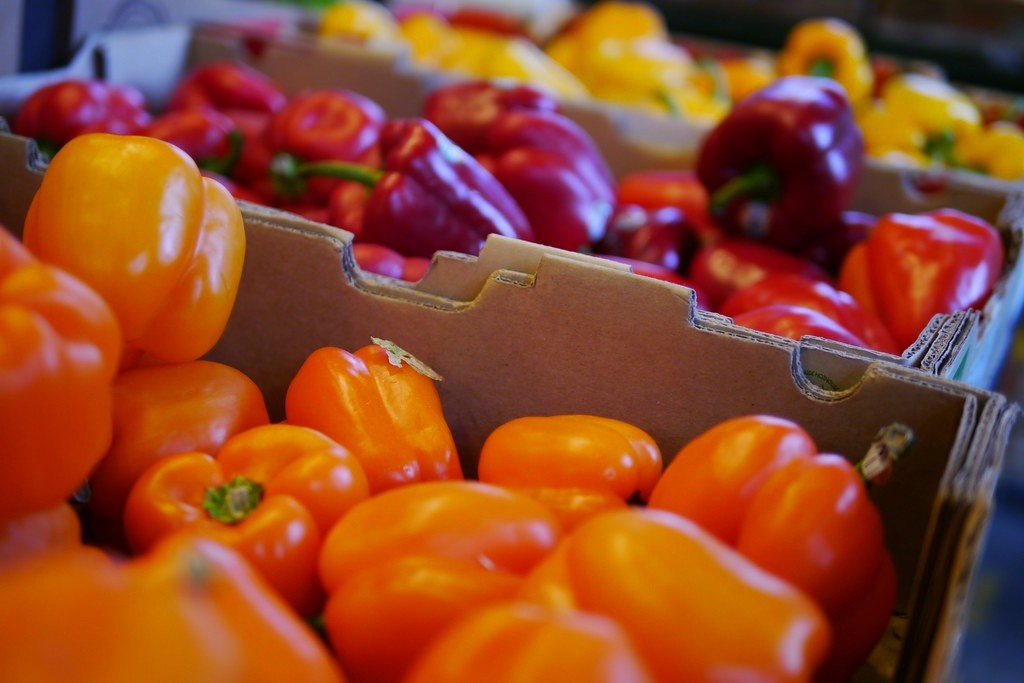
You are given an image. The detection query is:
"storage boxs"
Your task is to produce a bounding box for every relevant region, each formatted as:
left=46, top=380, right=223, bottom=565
left=0, top=0, right=1024, bottom=683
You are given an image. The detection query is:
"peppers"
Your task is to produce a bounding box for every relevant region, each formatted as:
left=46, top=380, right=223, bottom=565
left=0, top=0, right=1024, bottom=683
